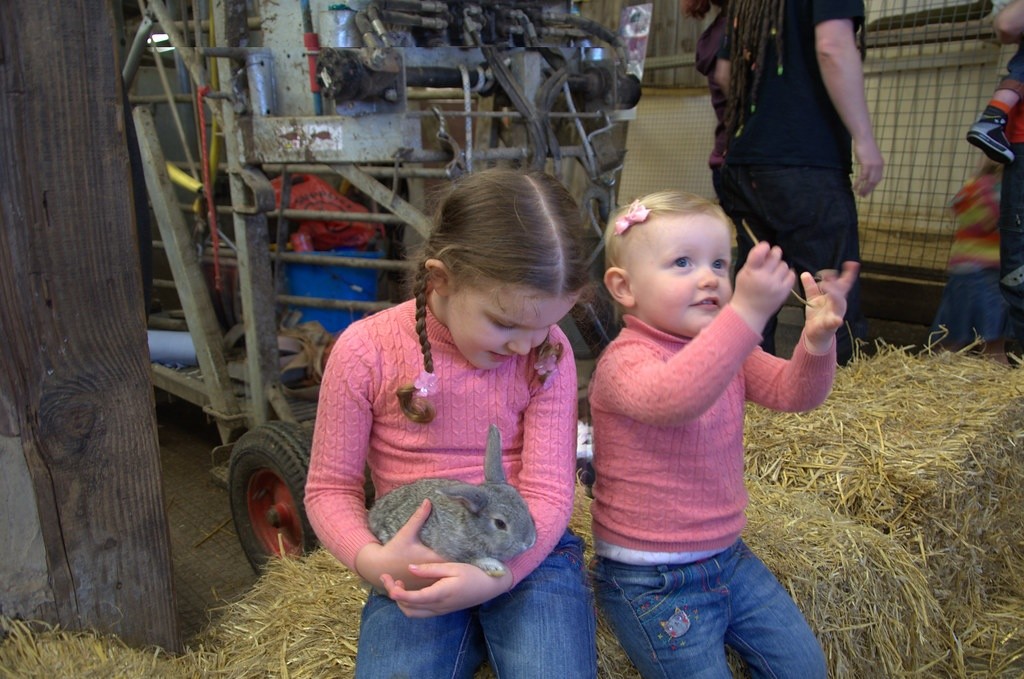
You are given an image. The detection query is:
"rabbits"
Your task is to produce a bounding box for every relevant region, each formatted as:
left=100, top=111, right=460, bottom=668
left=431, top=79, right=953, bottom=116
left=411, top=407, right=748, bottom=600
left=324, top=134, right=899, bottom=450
left=365, top=421, right=540, bottom=581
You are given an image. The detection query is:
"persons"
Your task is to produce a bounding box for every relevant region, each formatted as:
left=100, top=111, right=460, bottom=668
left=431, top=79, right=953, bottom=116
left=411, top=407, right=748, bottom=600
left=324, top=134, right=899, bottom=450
left=721, top=0, right=883, bottom=366
left=303, top=168, right=590, bottom=679
left=589, top=190, right=861, bottom=679
left=932, top=162, right=1000, bottom=359
left=682, top=0, right=731, bottom=203
left=988, top=0, right=1024, bottom=365
left=966, top=39, right=1024, bottom=163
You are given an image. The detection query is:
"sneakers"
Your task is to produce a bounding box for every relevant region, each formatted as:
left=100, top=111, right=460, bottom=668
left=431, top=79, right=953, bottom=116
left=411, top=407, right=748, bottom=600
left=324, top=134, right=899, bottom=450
left=966, top=113, right=1016, bottom=164
